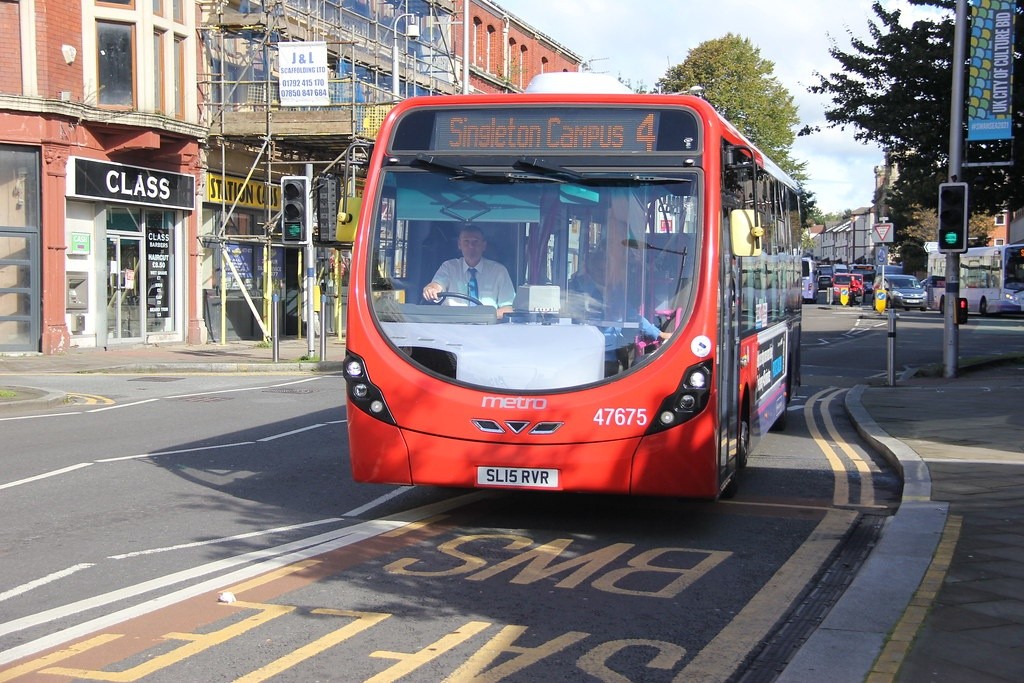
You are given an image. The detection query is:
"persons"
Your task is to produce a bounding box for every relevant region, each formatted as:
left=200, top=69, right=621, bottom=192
left=848, top=275, right=863, bottom=307
left=569, top=237, right=674, bottom=378
left=422, top=224, right=516, bottom=319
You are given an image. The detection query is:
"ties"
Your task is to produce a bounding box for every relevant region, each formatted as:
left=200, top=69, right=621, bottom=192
left=467, top=268, right=478, bottom=307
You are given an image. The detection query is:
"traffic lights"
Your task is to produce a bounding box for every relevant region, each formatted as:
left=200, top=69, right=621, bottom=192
left=281, top=176, right=309, bottom=244
left=939, top=182, right=968, bottom=253
left=954, top=297, right=969, bottom=324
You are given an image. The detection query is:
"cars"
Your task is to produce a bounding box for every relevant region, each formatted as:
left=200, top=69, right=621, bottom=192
left=817, top=259, right=927, bottom=311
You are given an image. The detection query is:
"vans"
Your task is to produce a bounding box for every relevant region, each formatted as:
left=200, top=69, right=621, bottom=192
left=800, top=256, right=818, bottom=303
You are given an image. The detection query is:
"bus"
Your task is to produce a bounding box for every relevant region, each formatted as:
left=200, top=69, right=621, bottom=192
left=927, top=244, right=1024, bottom=314
left=337, top=72, right=810, bottom=497
